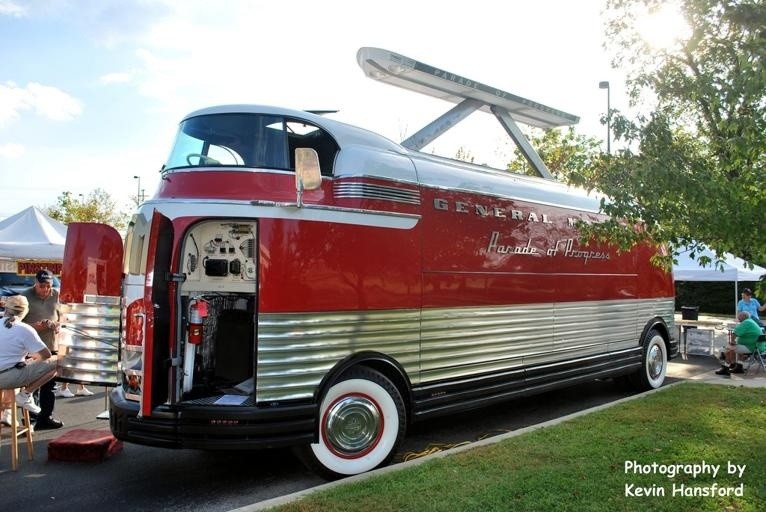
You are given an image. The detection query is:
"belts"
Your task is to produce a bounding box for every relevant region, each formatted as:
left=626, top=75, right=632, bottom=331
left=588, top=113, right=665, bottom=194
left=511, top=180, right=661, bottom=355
left=0, top=361, right=27, bottom=375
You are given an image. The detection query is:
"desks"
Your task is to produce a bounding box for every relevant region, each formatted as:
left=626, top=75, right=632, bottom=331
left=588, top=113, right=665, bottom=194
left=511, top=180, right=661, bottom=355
left=675, top=320, right=723, bottom=361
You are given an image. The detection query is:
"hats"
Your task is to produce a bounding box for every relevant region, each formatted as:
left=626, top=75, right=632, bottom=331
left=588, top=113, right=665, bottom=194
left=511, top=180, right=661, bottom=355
left=3, top=294, right=29, bottom=313
left=34, top=268, right=52, bottom=283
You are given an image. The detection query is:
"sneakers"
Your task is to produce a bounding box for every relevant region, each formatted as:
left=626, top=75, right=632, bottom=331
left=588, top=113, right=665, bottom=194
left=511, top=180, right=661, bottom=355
left=12, top=391, right=42, bottom=415
left=714, top=365, right=730, bottom=378
left=53, top=386, right=94, bottom=398
left=0, top=409, right=20, bottom=430
left=32, top=416, right=64, bottom=432
left=728, top=363, right=744, bottom=374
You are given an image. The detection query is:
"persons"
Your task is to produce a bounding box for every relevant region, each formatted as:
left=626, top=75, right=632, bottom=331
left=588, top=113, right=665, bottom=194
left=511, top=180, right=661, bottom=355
left=0, top=293, right=59, bottom=428
left=19, top=268, right=65, bottom=430
left=734, top=287, right=766, bottom=365
left=715, top=310, right=766, bottom=375
left=59, top=381, right=95, bottom=398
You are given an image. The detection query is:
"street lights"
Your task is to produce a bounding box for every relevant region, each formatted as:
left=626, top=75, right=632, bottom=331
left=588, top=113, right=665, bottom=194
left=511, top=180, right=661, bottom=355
left=599, top=81, right=610, bottom=153
left=134, top=176, right=139, bottom=205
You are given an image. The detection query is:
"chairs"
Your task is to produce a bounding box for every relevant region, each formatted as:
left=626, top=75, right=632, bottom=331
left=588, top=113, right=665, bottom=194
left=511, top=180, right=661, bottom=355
left=743, top=335, right=766, bottom=378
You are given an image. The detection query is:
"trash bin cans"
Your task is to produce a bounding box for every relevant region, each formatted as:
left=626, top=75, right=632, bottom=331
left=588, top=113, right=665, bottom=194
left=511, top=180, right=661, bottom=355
left=682, top=305, right=699, bottom=333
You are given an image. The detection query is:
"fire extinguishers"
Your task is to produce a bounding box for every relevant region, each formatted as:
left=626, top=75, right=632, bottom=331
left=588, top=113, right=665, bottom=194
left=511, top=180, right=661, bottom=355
left=188, top=293, right=210, bottom=345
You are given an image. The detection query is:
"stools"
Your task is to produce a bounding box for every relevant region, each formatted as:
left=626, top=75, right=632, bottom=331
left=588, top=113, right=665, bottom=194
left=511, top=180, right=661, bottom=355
left=0, top=386, right=34, bottom=471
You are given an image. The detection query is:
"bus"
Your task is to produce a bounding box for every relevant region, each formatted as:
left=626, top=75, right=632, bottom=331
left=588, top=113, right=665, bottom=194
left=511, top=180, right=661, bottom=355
left=56, top=45, right=679, bottom=482
